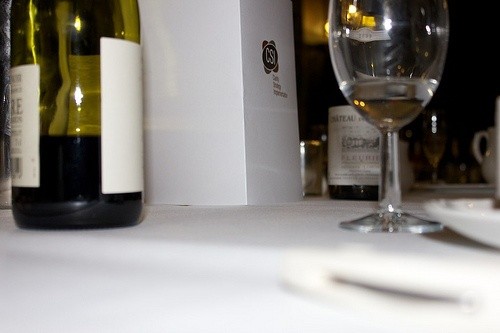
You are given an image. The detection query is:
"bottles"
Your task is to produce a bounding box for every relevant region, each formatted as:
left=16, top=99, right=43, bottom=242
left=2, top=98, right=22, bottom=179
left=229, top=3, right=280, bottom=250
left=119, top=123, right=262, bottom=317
left=328, top=49, right=380, bottom=202
left=9, top=0, right=145, bottom=228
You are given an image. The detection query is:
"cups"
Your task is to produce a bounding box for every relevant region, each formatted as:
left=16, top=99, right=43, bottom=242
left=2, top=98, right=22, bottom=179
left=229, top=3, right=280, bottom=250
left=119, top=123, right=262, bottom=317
left=300, top=141, right=323, bottom=197
left=474, top=129, right=496, bottom=185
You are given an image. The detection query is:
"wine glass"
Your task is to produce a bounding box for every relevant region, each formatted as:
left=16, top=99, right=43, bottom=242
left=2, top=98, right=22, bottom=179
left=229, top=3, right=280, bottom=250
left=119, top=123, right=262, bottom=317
left=327, top=0, right=446, bottom=234
